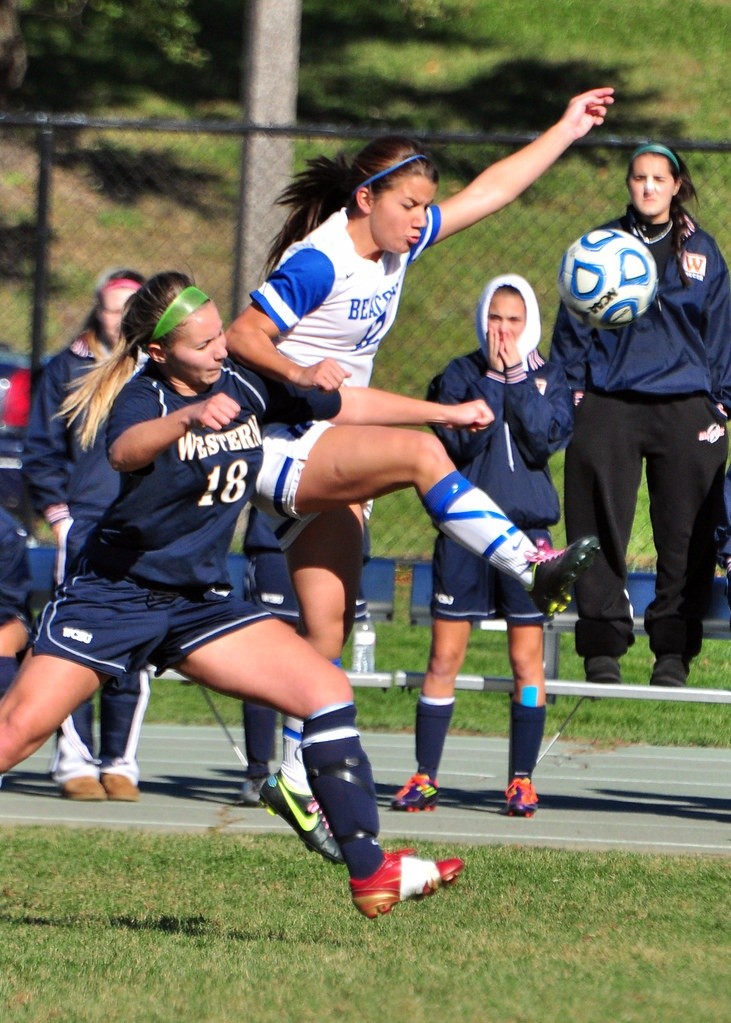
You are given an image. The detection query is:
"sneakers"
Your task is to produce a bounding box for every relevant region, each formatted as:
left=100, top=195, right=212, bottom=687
left=392, top=772, right=438, bottom=812
left=258, top=770, right=345, bottom=865
left=243, top=778, right=262, bottom=803
left=584, top=656, right=621, bottom=682
left=523, top=535, right=600, bottom=619
left=349, top=849, right=465, bottom=918
left=506, top=777, right=538, bottom=816
left=650, top=654, right=686, bottom=687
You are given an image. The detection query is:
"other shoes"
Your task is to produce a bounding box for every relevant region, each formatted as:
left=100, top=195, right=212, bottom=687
left=102, top=772, right=140, bottom=802
left=63, top=776, right=107, bottom=800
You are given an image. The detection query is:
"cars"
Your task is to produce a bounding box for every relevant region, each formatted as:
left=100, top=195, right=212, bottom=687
left=0, top=342, right=57, bottom=538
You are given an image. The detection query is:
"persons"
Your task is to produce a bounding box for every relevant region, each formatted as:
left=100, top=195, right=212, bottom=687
left=550, top=139, right=731, bottom=680
left=227, top=88, right=618, bottom=866
left=1, top=275, right=600, bottom=920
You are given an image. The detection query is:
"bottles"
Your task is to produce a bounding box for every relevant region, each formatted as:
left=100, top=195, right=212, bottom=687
left=351, top=611, right=375, bottom=674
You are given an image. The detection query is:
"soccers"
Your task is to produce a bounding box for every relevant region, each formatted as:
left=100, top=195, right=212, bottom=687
left=556, top=229, right=658, bottom=330
